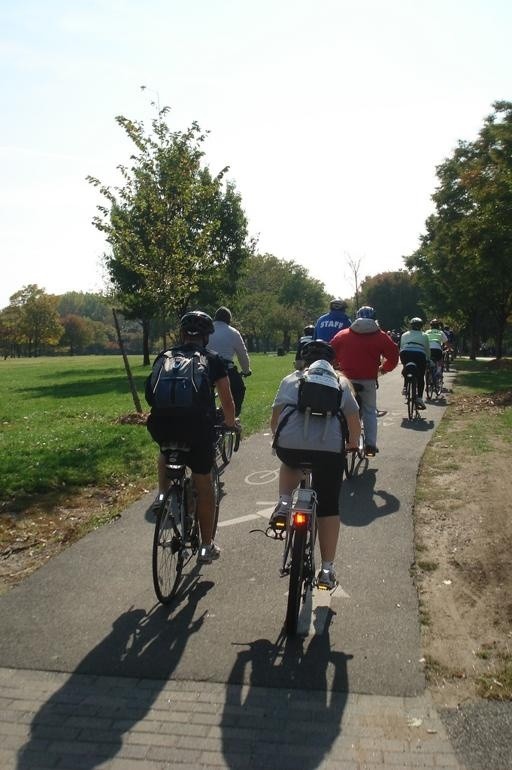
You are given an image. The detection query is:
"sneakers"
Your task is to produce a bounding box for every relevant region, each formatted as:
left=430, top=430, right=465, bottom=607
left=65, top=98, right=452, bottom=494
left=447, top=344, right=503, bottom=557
left=197, top=543, right=221, bottom=564
left=269, top=502, right=291, bottom=526
left=400, top=387, right=407, bottom=395
left=316, top=569, right=337, bottom=590
left=365, top=445, right=375, bottom=456
left=416, top=398, right=426, bottom=408
left=152, top=500, right=173, bottom=521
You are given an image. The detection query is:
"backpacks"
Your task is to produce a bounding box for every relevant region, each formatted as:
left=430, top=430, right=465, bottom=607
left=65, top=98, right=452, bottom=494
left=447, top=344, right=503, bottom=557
left=296, top=359, right=343, bottom=419
left=150, top=346, right=217, bottom=425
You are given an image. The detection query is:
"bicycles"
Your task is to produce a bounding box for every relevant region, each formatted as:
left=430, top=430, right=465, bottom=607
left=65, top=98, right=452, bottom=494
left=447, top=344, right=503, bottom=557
left=191, top=370, right=251, bottom=492
left=271, top=433, right=364, bottom=636
left=343, top=367, right=387, bottom=480
left=401, top=342, right=453, bottom=422
left=153, top=422, right=242, bottom=604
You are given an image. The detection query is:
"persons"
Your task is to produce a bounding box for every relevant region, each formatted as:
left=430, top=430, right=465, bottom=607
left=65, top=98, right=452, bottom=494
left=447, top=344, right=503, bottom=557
left=481, top=341, right=488, bottom=357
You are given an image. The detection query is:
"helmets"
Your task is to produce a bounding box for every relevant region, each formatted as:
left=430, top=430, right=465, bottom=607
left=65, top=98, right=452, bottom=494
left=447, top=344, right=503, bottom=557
left=356, top=305, right=377, bottom=319
left=430, top=318, right=438, bottom=327
left=302, top=324, right=315, bottom=334
left=330, top=298, right=348, bottom=310
left=302, top=338, right=335, bottom=362
left=180, top=310, right=215, bottom=334
left=410, top=317, right=423, bottom=326
